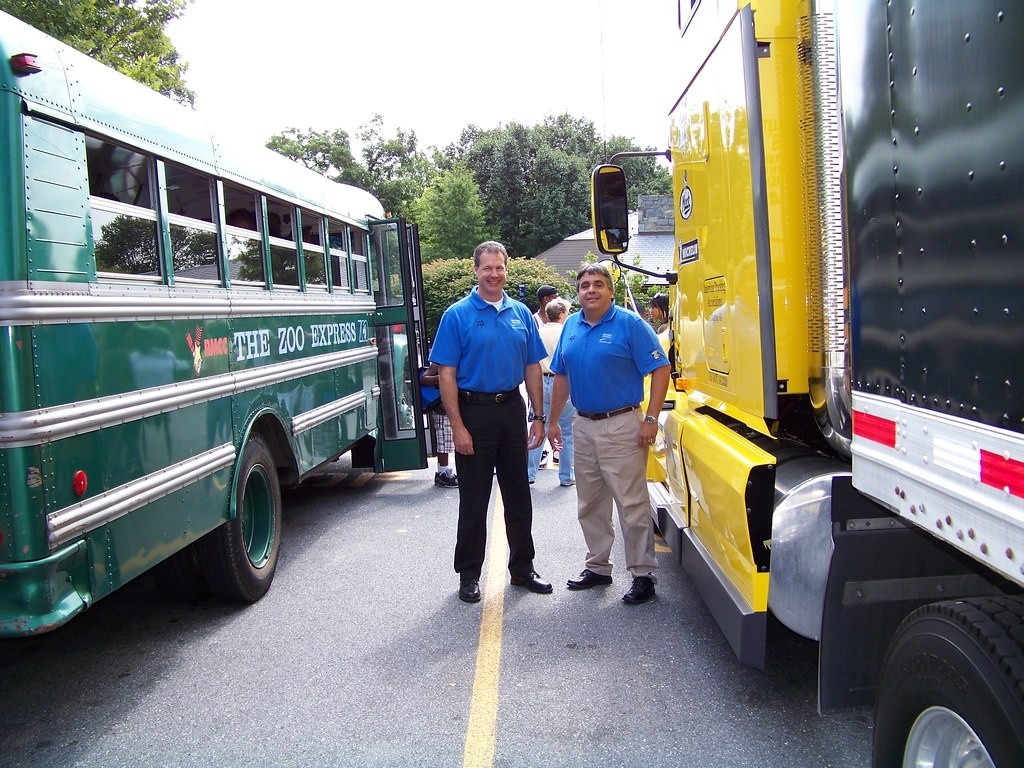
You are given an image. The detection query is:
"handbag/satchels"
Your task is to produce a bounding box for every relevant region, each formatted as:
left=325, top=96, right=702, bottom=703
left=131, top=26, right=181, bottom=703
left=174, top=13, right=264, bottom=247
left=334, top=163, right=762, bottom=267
left=406, top=369, right=441, bottom=414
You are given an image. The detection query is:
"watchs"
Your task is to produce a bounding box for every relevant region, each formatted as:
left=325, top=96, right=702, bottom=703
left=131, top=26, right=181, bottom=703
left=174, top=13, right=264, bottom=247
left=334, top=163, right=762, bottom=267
left=534, top=413, right=546, bottom=423
left=645, top=416, right=658, bottom=423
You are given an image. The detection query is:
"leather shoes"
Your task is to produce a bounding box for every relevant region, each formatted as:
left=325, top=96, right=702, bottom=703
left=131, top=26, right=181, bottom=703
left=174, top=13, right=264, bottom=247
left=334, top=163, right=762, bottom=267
left=509, top=571, right=553, bottom=593
left=566, top=570, right=613, bottom=589
left=621, top=576, right=655, bottom=604
left=458, top=578, right=482, bottom=603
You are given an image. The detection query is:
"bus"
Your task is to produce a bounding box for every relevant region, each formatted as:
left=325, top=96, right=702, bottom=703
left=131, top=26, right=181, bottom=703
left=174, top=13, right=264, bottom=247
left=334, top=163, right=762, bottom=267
left=0, top=10, right=432, bottom=640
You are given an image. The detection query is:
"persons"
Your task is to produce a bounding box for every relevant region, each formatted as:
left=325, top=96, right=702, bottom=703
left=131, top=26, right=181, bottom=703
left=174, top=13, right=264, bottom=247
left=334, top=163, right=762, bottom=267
left=228, top=208, right=257, bottom=232
left=528, top=285, right=578, bottom=486
left=268, top=212, right=287, bottom=239
left=302, top=225, right=313, bottom=243
left=649, top=292, right=669, bottom=334
left=427, top=241, right=553, bottom=603
left=420, top=361, right=460, bottom=488
left=546, top=263, right=672, bottom=604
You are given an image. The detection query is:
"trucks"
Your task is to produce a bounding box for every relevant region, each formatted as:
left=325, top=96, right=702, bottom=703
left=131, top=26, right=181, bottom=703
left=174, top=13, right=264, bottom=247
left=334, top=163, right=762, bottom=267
left=587, top=0, right=1023, bottom=768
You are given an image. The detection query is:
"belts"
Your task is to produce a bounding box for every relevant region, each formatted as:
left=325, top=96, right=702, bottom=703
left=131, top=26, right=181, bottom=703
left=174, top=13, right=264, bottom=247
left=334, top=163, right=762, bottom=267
left=543, top=373, right=556, bottom=377
left=573, top=402, right=641, bottom=420
left=457, top=386, right=520, bottom=403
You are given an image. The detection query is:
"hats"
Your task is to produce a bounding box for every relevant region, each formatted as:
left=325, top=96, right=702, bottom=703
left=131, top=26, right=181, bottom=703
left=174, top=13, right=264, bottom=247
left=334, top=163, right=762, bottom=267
left=536, top=285, right=558, bottom=301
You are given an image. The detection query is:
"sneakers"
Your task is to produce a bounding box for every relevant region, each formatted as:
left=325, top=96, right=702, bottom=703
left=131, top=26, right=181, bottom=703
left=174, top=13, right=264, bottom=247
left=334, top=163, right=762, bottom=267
left=560, top=479, right=576, bottom=486
left=434, top=471, right=459, bottom=488
left=529, top=479, right=535, bottom=484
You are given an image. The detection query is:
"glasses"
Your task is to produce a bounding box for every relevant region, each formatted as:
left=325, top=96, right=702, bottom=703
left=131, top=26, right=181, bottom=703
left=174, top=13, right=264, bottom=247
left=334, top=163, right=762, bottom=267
left=653, top=292, right=661, bottom=299
